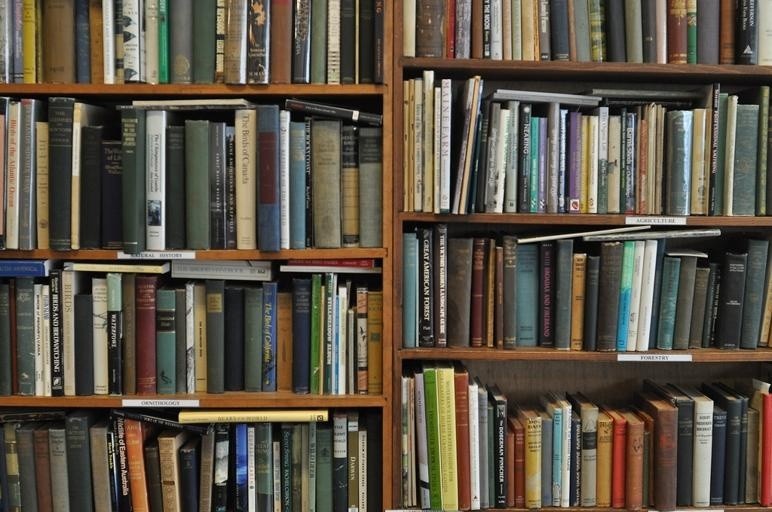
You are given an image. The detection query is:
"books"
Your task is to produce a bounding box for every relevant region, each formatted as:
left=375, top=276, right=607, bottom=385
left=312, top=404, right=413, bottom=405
left=4, top=2, right=384, bottom=85
left=3, top=259, right=384, bottom=395
left=396, top=359, right=771, bottom=510
left=402, top=70, right=771, bottom=217
left=400, top=1, right=771, bottom=68
left=402, top=227, right=771, bottom=351
left=3, top=409, right=382, bottom=510
left=3, top=96, right=383, bottom=251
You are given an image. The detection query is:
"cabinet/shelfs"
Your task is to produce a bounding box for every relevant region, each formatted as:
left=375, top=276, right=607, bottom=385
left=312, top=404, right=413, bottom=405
left=0, top=1, right=390, bottom=512
left=397, top=0, right=772, bottom=512
